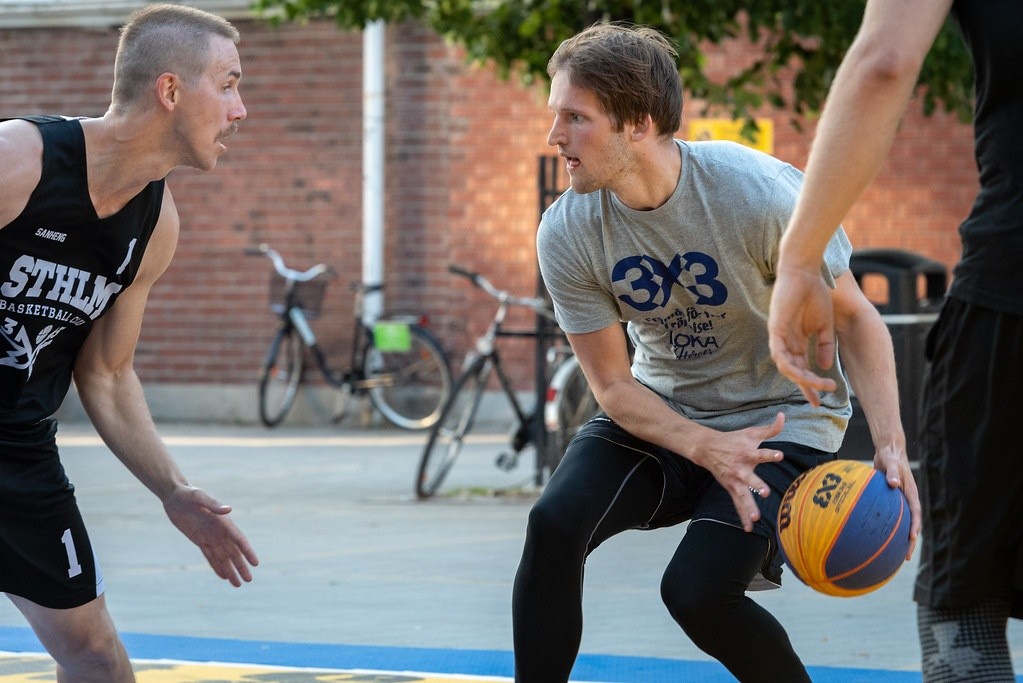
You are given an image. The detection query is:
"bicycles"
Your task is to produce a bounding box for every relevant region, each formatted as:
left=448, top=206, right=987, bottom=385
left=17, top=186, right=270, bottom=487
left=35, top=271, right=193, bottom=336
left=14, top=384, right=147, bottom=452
left=543, top=342, right=598, bottom=476
left=243, top=243, right=453, bottom=432
left=414, top=265, right=572, bottom=501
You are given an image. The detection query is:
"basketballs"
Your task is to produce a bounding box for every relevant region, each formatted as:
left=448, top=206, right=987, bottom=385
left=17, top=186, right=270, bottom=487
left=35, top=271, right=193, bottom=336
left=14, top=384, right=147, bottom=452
left=775, top=460, right=912, bottom=598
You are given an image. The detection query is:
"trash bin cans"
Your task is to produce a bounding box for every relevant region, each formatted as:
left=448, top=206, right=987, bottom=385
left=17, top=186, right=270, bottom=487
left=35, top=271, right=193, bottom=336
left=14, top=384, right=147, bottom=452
left=836, top=246, right=951, bottom=480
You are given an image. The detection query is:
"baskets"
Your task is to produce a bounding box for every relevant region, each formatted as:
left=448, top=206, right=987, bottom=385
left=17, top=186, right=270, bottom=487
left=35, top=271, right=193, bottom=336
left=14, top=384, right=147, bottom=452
left=266, top=272, right=326, bottom=319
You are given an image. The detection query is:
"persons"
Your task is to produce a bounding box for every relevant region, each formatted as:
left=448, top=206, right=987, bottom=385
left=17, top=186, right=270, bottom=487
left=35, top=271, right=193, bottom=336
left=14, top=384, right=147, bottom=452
left=511, top=24, right=920, bottom=683
left=767, top=0, right=1023, bottom=683
left=0, top=3, right=260, bottom=683
